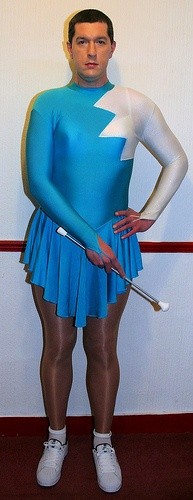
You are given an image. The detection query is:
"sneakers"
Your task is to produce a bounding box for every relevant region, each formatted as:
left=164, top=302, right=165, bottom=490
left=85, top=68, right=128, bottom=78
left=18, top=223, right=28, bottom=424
left=92, top=443, right=122, bottom=492
left=37, top=437, right=69, bottom=486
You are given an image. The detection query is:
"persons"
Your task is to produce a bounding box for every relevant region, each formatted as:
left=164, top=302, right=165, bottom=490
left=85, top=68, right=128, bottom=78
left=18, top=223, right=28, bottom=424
left=19, top=10, right=188, bottom=493
